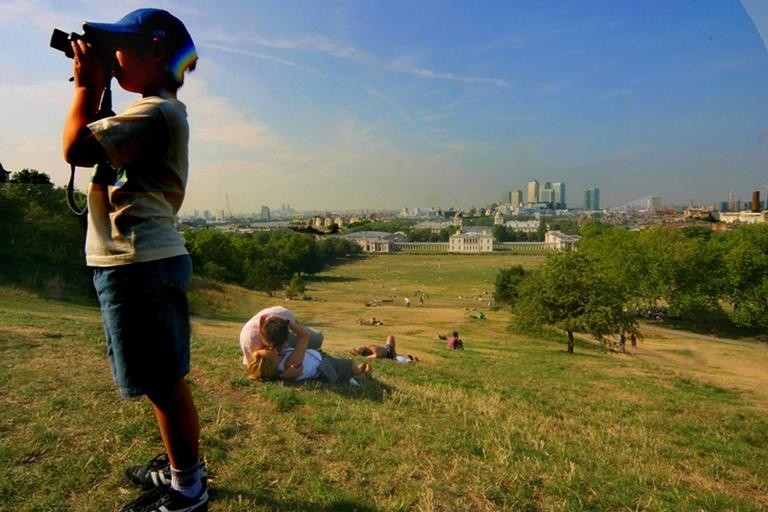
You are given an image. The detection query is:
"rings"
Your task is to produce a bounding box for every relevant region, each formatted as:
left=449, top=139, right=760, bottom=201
left=291, top=364, right=294, bottom=367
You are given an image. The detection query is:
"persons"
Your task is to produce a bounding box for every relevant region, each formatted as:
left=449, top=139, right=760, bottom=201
left=404, top=295, right=410, bottom=307
left=244, top=347, right=373, bottom=383
left=619, top=330, right=627, bottom=351
left=446, top=330, right=464, bottom=350
left=628, top=332, right=636, bottom=350
left=48, top=9, right=212, bottom=510
left=239, top=305, right=323, bottom=380
left=645, top=305, right=667, bottom=322
left=349, top=335, right=396, bottom=360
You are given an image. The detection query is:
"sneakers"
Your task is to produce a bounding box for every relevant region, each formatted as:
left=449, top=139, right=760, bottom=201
left=125, top=453, right=211, bottom=494
left=118, top=475, right=210, bottom=512
left=357, top=362, right=372, bottom=381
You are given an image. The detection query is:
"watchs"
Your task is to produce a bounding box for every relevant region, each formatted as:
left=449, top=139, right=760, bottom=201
left=280, top=373, right=286, bottom=380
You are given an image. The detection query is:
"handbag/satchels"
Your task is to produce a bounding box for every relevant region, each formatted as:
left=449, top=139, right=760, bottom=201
left=316, top=358, right=339, bottom=385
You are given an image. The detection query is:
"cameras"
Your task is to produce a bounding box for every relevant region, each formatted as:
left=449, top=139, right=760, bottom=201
left=49, top=27, right=115, bottom=72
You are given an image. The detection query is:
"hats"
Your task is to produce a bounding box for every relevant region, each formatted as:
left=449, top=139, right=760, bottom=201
left=78, top=6, right=199, bottom=69
